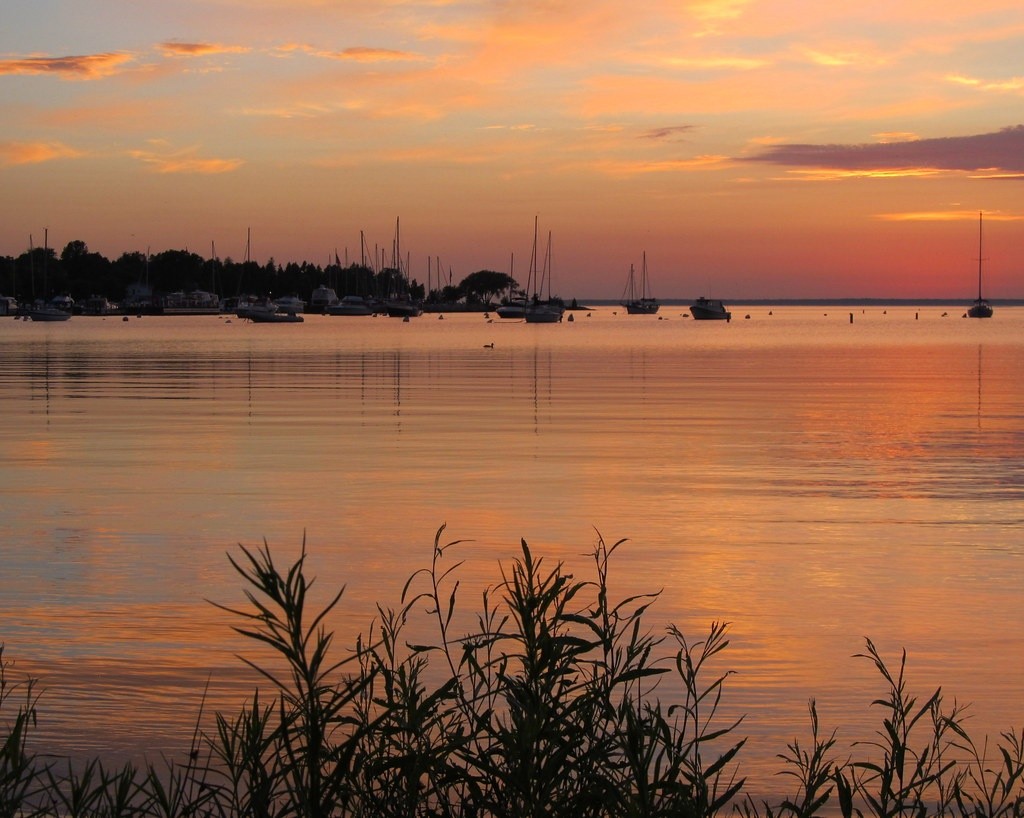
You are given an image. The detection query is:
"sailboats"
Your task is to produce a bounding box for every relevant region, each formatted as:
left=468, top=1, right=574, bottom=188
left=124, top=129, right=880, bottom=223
left=968, top=213, right=994, bottom=319
left=526, top=215, right=562, bottom=323
left=536, top=230, right=565, bottom=315
left=0, top=215, right=452, bottom=321
left=619, top=251, right=661, bottom=314
left=496, top=252, right=532, bottom=318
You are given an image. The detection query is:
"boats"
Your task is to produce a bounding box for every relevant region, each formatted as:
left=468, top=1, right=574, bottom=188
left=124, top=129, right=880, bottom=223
left=689, top=298, right=731, bottom=321
left=250, top=312, right=304, bottom=323
